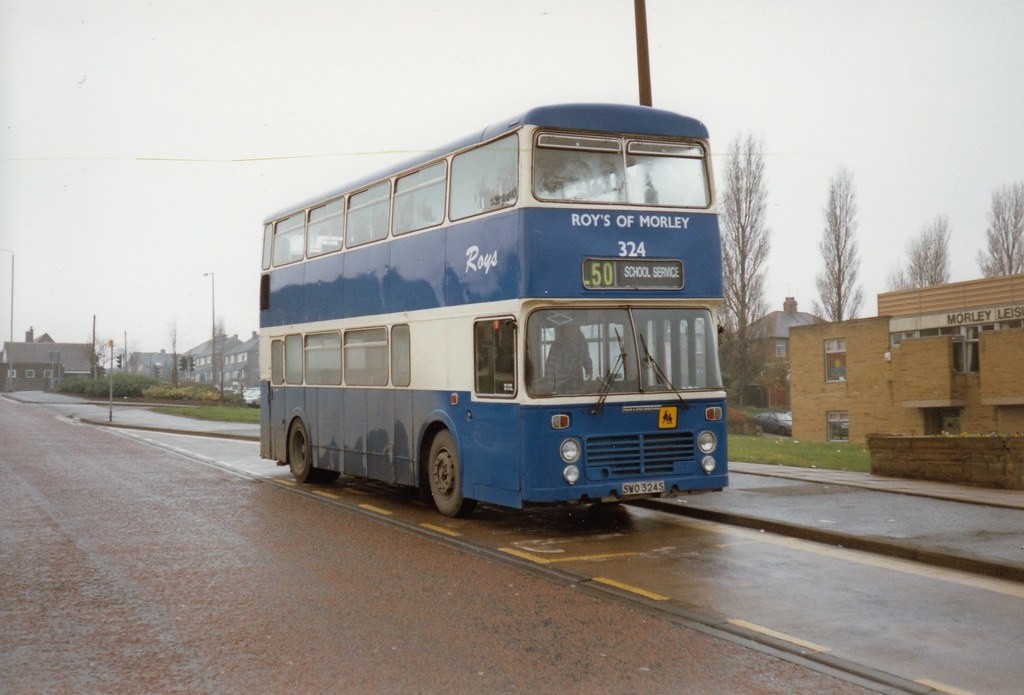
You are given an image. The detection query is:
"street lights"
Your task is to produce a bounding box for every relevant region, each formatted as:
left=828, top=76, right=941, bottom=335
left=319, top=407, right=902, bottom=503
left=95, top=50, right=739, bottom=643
left=204, top=271, right=216, bottom=388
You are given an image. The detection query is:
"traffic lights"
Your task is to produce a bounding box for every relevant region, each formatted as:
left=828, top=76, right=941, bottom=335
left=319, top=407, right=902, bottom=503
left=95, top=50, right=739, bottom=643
left=190, top=356, right=195, bottom=372
left=116, top=354, right=122, bottom=369
left=178, top=355, right=186, bottom=372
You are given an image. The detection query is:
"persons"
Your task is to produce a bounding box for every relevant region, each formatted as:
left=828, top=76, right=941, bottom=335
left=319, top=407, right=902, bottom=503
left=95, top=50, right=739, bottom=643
left=544, top=326, right=593, bottom=381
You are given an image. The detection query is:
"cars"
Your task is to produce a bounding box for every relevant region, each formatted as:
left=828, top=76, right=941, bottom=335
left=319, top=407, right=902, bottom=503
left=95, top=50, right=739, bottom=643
left=754, top=412, right=793, bottom=437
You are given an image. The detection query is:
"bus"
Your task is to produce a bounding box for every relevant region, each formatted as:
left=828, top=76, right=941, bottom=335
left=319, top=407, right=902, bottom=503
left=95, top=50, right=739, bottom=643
left=259, top=102, right=729, bottom=519
